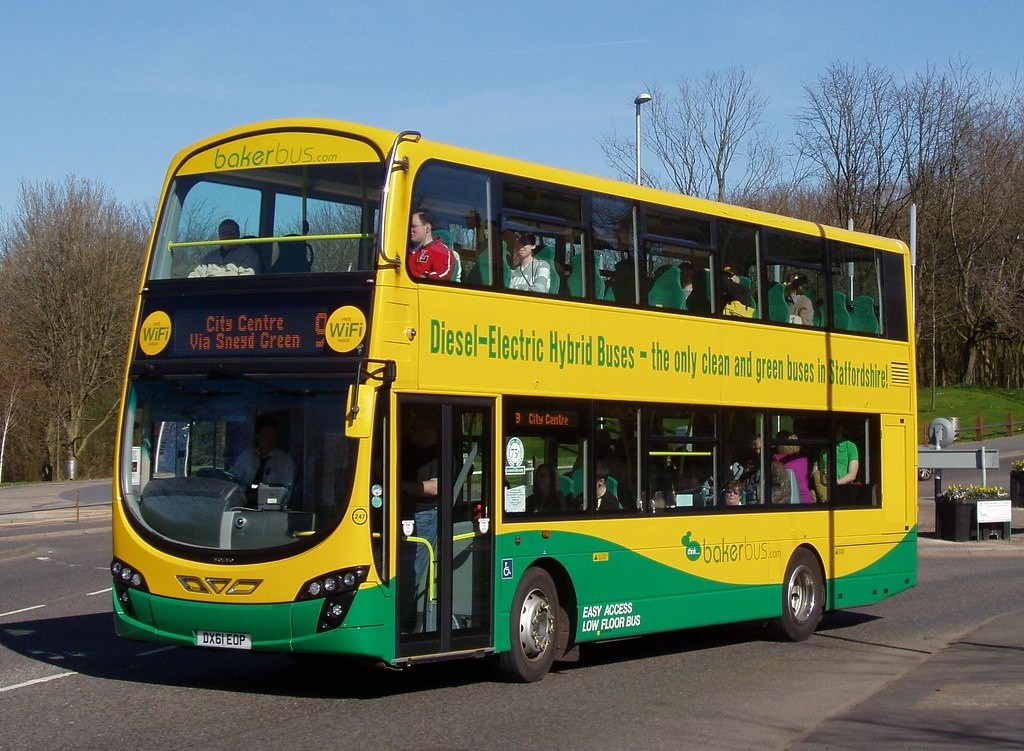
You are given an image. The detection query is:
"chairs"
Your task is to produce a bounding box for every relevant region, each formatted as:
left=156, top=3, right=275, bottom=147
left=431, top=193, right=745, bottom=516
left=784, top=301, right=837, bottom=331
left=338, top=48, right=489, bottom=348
left=467, top=239, right=511, bottom=290
left=804, top=286, right=851, bottom=332
left=612, top=257, right=645, bottom=307
left=240, top=233, right=312, bottom=273
left=684, top=269, right=713, bottom=316
left=431, top=229, right=459, bottom=282
left=558, top=466, right=748, bottom=510
left=728, top=274, right=756, bottom=319
left=643, top=264, right=688, bottom=312
left=532, top=245, right=560, bottom=295
left=564, top=250, right=605, bottom=301
left=785, top=468, right=802, bottom=504
left=762, top=282, right=791, bottom=326
left=290, top=438, right=318, bottom=515
left=850, top=294, right=881, bottom=334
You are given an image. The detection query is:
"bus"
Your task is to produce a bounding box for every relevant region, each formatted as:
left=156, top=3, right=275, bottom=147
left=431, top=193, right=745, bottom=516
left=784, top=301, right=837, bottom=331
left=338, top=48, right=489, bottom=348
left=109, top=118, right=918, bottom=687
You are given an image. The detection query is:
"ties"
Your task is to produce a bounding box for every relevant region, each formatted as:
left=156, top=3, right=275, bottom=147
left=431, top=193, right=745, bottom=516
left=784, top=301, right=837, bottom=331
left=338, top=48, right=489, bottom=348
left=251, top=455, right=271, bottom=495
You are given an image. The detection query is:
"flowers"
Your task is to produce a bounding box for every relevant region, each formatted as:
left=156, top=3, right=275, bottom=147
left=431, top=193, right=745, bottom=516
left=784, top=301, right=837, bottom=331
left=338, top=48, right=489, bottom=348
left=1010, top=458, right=1024, bottom=472
left=935, top=483, right=1010, bottom=499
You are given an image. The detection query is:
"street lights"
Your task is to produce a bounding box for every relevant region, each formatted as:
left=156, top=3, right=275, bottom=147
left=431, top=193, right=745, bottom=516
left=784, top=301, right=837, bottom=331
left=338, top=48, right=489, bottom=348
left=633, top=94, right=653, bottom=186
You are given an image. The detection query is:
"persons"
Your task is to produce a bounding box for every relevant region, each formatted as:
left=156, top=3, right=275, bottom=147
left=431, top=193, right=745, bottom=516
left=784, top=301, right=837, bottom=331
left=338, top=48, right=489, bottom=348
left=571, top=463, right=619, bottom=510
left=525, top=463, right=572, bottom=516
left=574, top=429, right=613, bottom=470
left=398, top=403, right=439, bottom=635
left=201, top=218, right=259, bottom=274
left=646, top=429, right=859, bottom=508
left=226, top=413, right=296, bottom=509
left=408, top=209, right=456, bottom=281
left=613, top=258, right=815, bottom=325
left=462, top=209, right=571, bottom=295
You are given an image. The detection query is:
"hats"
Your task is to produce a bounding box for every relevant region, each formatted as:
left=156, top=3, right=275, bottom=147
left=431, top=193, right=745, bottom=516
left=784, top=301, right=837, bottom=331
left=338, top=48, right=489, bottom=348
left=722, top=263, right=738, bottom=276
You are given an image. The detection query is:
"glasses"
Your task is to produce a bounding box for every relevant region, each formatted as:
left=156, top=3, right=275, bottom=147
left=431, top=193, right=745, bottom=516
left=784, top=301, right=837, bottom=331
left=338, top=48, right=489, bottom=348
left=728, top=273, right=736, bottom=278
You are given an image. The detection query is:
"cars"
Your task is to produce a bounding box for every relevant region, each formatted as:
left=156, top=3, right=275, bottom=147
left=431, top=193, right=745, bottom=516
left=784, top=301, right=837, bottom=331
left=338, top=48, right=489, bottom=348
left=917, top=445, right=935, bottom=482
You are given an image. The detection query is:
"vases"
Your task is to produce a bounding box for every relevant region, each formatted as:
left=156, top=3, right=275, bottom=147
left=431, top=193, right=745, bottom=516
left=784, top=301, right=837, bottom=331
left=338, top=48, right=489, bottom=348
left=1010, top=471, right=1024, bottom=508
left=934, top=496, right=1009, bottom=542
